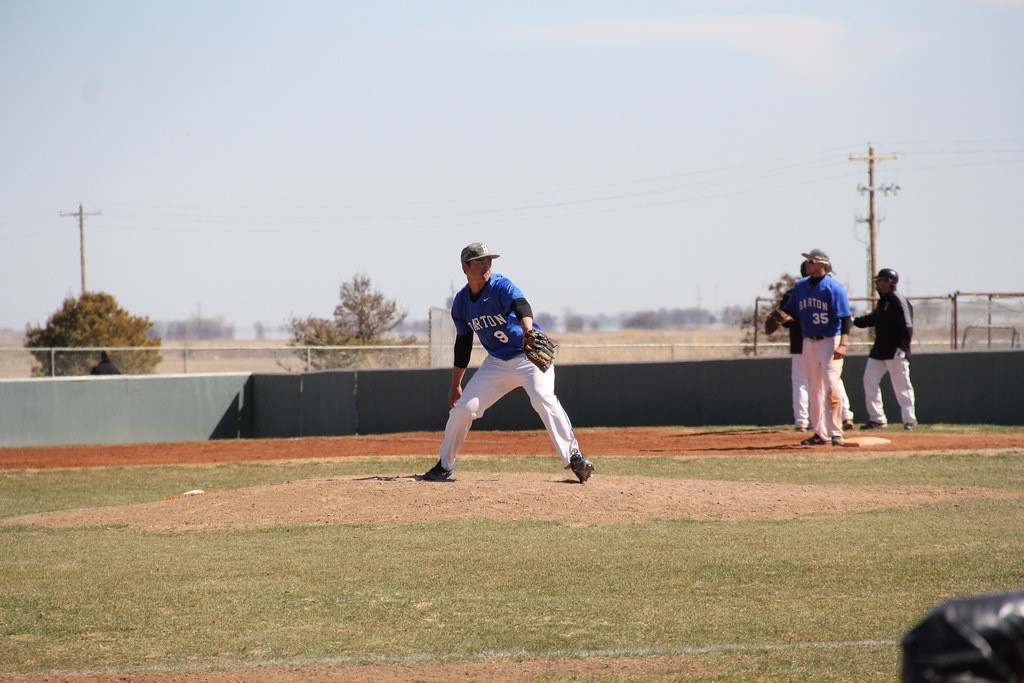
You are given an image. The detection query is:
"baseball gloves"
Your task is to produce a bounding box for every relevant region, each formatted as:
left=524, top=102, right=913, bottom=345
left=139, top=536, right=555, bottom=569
left=764, top=310, right=795, bottom=335
left=522, top=327, right=559, bottom=374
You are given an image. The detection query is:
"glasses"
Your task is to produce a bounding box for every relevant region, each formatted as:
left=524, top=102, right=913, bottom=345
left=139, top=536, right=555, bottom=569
left=805, top=259, right=825, bottom=264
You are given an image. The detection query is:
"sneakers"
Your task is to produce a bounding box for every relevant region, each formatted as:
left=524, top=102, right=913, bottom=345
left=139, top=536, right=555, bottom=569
left=801, top=432, right=830, bottom=445
left=570, top=455, right=594, bottom=483
left=833, top=434, right=845, bottom=447
left=424, top=459, right=454, bottom=481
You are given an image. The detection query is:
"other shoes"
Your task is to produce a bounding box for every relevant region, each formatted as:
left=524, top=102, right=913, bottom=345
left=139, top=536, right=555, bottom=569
left=841, top=417, right=853, bottom=431
left=861, top=419, right=886, bottom=430
left=905, top=423, right=917, bottom=431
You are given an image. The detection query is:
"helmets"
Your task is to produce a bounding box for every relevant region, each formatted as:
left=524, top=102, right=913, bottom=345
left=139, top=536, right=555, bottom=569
left=872, top=269, right=898, bottom=282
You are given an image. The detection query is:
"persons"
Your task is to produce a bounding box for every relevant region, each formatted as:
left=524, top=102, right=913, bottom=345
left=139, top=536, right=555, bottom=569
left=849, top=269, right=918, bottom=430
left=765, top=248, right=854, bottom=445
left=91, top=350, right=123, bottom=374
left=421, top=241, right=594, bottom=482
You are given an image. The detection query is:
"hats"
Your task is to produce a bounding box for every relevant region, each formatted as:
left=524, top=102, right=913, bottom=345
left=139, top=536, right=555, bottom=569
left=801, top=248, right=829, bottom=264
left=461, top=243, right=500, bottom=262
